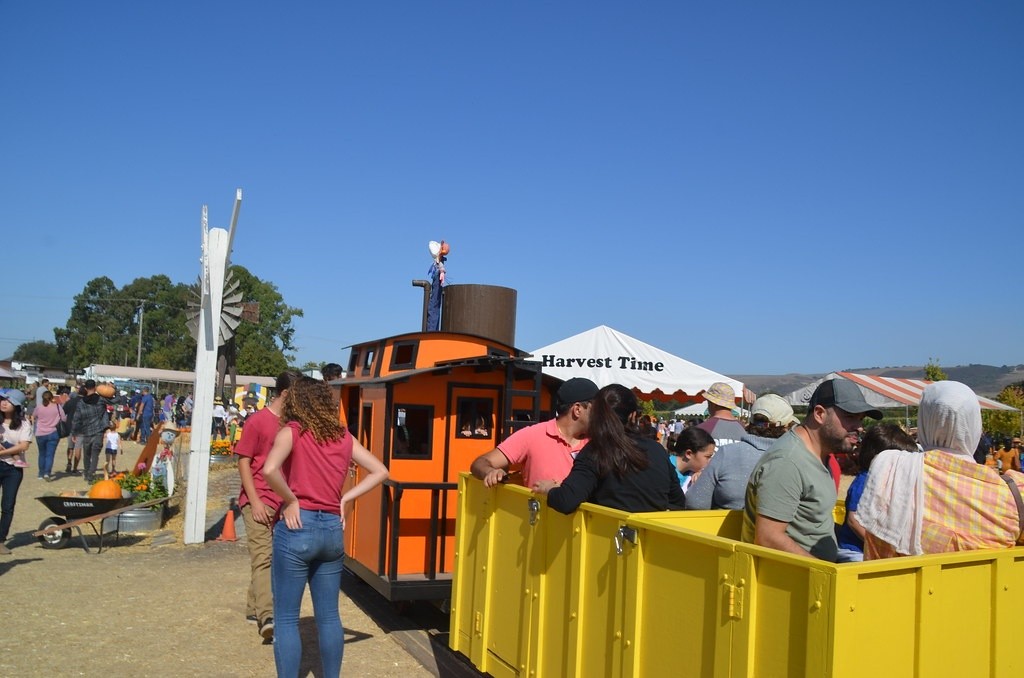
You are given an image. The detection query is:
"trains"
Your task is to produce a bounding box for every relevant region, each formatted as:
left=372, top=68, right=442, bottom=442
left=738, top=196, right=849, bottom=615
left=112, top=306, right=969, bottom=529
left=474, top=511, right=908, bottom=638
left=328, top=279, right=1024, bottom=678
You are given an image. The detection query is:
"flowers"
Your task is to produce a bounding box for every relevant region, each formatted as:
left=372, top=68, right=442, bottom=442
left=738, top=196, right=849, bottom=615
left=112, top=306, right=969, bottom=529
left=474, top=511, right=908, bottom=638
left=210, top=437, right=237, bottom=455
left=96, top=465, right=168, bottom=506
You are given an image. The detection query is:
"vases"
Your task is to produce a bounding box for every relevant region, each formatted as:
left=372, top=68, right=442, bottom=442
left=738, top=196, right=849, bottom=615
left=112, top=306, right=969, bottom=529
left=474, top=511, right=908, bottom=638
left=100, top=502, right=166, bottom=533
left=209, top=453, right=240, bottom=466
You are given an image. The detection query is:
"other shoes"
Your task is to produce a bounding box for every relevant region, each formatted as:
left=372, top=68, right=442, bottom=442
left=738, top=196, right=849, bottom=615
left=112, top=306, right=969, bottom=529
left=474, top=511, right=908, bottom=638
left=0, top=543, right=12, bottom=554
left=260, top=617, right=274, bottom=638
left=65, top=464, right=72, bottom=472
left=246, top=614, right=258, bottom=624
left=44, top=474, right=49, bottom=482
left=72, top=470, right=81, bottom=475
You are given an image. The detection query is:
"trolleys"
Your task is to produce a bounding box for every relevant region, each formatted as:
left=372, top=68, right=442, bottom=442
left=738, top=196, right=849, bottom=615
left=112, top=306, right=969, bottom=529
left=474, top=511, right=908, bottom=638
left=32, top=493, right=182, bottom=555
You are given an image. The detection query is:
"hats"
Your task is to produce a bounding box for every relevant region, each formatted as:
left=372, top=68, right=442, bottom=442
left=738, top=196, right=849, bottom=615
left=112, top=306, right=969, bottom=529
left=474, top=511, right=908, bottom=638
left=807, top=378, right=883, bottom=421
left=214, top=396, right=224, bottom=405
left=750, top=394, right=801, bottom=427
left=556, top=377, right=600, bottom=405
left=701, top=382, right=737, bottom=409
left=0, top=389, right=27, bottom=417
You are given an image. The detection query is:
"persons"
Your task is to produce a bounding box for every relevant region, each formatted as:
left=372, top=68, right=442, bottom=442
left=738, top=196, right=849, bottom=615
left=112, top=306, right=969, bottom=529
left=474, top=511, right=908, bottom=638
left=321, top=363, right=343, bottom=383
left=636, top=378, right=924, bottom=564
left=531, top=384, right=686, bottom=515
left=0, top=389, right=34, bottom=554
left=234, top=368, right=306, bottom=637
left=973, top=426, right=1024, bottom=475
left=470, top=378, right=600, bottom=488
left=26, top=378, right=193, bottom=485
left=263, top=376, right=390, bottom=678
left=0, top=412, right=29, bottom=467
left=212, top=395, right=256, bottom=442
left=854, top=379, right=1024, bottom=562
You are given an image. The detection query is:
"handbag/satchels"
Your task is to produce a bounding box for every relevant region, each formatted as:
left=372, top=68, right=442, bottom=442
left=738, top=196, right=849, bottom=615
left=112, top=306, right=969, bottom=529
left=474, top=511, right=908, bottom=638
left=57, top=420, right=69, bottom=438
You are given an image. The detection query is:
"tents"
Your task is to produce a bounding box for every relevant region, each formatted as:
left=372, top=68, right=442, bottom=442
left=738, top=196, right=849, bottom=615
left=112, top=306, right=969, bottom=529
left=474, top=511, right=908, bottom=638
left=780, top=371, right=1020, bottom=429
left=524, top=325, right=756, bottom=424
